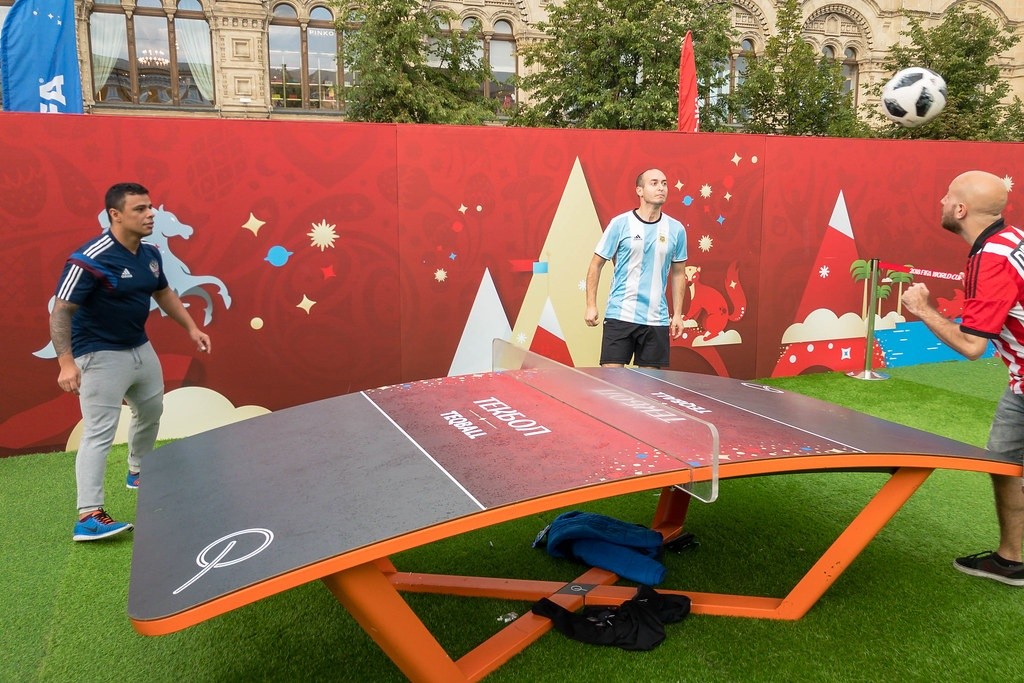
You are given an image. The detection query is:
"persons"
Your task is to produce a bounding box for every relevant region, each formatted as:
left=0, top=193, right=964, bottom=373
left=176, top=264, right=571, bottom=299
left=48, top=182, right=211, bottom=540
left=901, top=170, right=1024, bottom=586
left=584, top=168, right=687, bottom=368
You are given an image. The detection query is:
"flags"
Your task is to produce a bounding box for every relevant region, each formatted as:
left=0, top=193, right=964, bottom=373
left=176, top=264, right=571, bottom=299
left=0, top=0, right=85, bottom=114
left=678, top=30, right=701, bottom=133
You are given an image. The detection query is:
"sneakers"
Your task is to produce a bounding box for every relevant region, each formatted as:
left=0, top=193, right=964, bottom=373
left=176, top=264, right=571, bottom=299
left=72, top=507, right=135, bottom=541
left=953, top=550, right=1024, bottom=586
left=126, top=471, right=142, bottom=488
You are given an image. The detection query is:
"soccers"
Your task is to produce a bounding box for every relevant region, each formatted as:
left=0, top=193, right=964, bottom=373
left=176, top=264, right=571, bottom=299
left=881, top=66, right=947, bottom=129
